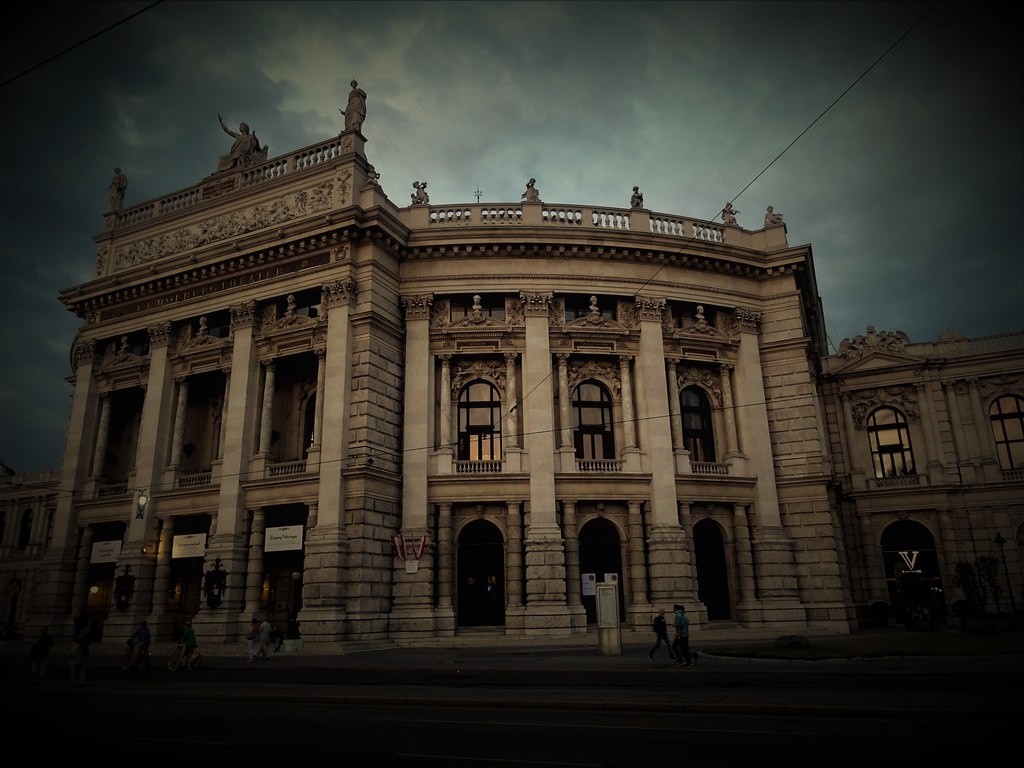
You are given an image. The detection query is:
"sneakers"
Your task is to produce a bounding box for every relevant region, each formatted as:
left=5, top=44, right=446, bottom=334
left=648, top=655, right=654, bottom=659
left=669, top=655, right=676, bottom=660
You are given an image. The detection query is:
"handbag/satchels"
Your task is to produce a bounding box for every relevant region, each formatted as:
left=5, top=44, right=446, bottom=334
left=246, top=630, right=256, bottom=640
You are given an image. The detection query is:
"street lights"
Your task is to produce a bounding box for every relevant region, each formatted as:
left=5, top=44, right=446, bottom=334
left=991, top=529, right=1019, bottom=635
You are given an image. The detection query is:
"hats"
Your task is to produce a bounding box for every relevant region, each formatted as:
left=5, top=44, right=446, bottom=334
left=673, top=604, right=681, bottom=610
left=184, top=621, right=192, bottom=626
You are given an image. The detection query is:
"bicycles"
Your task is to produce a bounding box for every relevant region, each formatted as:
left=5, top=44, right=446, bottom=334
left=120, top=636, right=153, bottom=673
left=167, top=639, right=203, bottom=670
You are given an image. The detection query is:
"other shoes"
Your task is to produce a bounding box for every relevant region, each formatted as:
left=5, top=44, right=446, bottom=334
left=262, top=656, right=269, bottom=659
left=248, top=658, right=253, bottom=661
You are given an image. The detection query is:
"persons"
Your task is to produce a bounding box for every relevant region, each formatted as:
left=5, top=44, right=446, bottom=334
left=196, top=316, right=209, bottom=336
left=526, top=178, right=541, bottom=202
left=107, top=167, right=128, bottom=211
left=250, top=616, right=284, bottom=656
left=630, top=186, right=644, bottom=209
left=215, top=113, right=268, bottom=174
left=339, top=77, right=367, bottom=134
left=120, top=336, right=128, bottom=344
left=472, top=294, right=482, bottom=309
left=765, top=206, right=784, bottom=224
left=68, top=612, right=96, bottom=688
left=175, top=621, right=196, bottom=666
left=695, top=306, right=705, bottom=319
left=589, top=296, right=599, bottom=310
left=648, top=605, right=691, bottom=666
left=411, top=181, right=429, bottom=205
left=287, top=295, right=296, bottom=303
left=722, top=202, right=740, bottom=225
left=35, top=626, right=53, bottom=690
left=130, top=621, right=150, bottom=662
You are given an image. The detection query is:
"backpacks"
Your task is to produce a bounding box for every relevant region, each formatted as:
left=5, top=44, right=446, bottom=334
left=653, top=616, right=662, bottom=633
